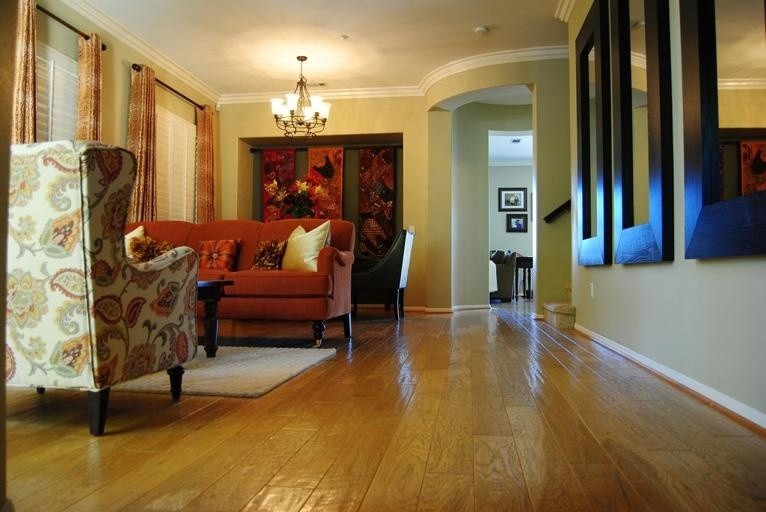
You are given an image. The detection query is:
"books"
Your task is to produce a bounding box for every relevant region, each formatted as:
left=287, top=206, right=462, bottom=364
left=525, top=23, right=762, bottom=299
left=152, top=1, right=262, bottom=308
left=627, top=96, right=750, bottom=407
left=530, top=313, right=544, bottom=320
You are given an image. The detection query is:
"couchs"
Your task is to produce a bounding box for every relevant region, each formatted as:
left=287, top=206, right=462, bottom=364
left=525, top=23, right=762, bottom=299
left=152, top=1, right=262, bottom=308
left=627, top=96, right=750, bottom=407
left=121, top=219, right=357, bottom=348
left=490, top=249, right=517, bottom=303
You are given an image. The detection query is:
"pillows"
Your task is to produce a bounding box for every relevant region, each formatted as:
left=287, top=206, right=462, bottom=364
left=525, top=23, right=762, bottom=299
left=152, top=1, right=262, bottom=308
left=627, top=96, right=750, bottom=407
left=124, top=225, right=145, bottom=259
left=249, top=238, right=288, bottom=271
left=281, top=220, right=332, bottom=273
left=198, top=239, right=241, bottom=272
left=129, top=235, right=173, bottom=264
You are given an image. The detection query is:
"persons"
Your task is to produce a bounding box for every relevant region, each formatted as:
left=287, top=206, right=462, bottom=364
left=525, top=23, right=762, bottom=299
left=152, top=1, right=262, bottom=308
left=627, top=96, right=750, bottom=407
left=515, top=220, right=521, bottom=228
left=509, top=193, right=519, bottom=205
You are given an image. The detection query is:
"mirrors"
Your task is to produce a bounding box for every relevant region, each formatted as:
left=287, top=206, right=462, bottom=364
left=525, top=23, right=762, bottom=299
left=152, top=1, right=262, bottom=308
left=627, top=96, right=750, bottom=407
left=608, top=0, right=675, bottom=265
left=678, top=0, right=766, bottom=260
left=574, top=0, right=612, bottom=268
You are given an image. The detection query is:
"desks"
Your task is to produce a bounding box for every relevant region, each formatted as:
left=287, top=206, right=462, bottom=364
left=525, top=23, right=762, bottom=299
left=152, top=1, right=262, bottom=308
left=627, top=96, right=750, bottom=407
left=513, top=256, right=533, bottom=302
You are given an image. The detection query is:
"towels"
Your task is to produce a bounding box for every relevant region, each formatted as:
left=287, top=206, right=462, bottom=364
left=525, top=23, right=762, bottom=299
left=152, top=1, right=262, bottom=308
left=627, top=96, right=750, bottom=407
left=489, top=260, right=499, bottom=294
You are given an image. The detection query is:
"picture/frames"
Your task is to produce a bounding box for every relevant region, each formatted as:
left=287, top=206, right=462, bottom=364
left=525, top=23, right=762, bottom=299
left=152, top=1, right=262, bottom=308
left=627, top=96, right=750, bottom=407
left=498, top=188, right=528, bottom=212
left=505, top=213, right=528, bottom=232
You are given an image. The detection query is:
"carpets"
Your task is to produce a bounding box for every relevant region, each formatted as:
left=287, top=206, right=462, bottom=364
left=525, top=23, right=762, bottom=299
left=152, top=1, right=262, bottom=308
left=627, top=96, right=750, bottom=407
left=110, top=343, right=338, bottom=399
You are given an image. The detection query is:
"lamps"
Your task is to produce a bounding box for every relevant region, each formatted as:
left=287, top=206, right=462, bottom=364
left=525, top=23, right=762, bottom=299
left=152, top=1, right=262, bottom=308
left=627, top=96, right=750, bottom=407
left=267, top=56, right=333, bottom=142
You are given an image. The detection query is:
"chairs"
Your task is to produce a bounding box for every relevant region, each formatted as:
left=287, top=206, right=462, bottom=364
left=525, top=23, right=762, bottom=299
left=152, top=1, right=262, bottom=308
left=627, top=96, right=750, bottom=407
left=3, top=138, right=200, bottom=436
left=351, top=229, right=415, bottom=321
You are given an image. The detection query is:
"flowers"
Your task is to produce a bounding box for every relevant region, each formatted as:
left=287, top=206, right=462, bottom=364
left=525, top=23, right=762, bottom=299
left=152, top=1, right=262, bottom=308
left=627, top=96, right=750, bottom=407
left=262, top=174, right=332, bottom=219
